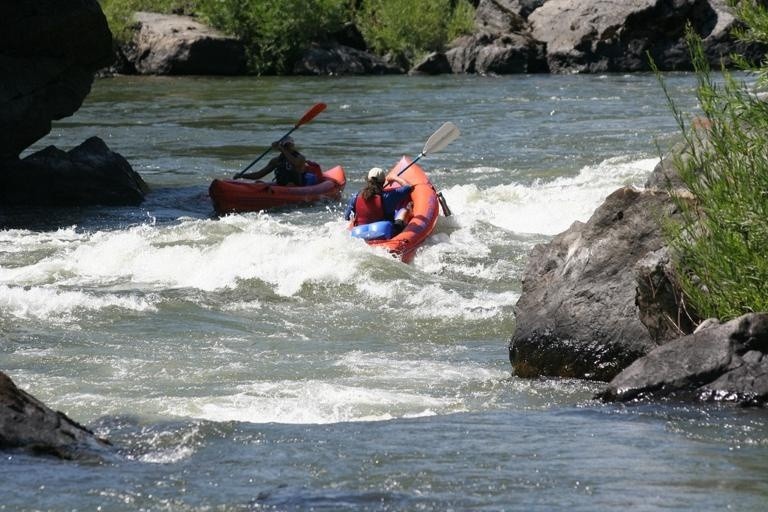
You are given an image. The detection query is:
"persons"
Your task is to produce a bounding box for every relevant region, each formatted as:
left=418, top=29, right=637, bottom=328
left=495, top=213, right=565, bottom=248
left=346, top=166, right=415, bottom=237
left=233, top=135, right=306, bottom=186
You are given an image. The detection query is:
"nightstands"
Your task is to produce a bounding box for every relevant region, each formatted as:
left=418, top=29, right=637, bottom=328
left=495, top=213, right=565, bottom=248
left=233, top=103, right=327, bottom=179
left=383, top=122, right=460, bottom=189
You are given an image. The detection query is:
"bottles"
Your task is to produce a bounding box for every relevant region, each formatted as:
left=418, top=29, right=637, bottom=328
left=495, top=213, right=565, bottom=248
left=367, top=167, right=385, bottom=183
left=281, top=136, right=294, bottom=146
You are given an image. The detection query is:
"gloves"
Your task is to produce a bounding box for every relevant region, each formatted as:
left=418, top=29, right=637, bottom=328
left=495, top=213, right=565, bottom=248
left=210, top=165, right=345, bottom=214
left=349, top=155, right=451, bottom=264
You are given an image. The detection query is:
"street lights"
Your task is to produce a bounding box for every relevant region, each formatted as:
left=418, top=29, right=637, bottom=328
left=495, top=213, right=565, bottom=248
left=303, top=160, right=321, bottom=181
